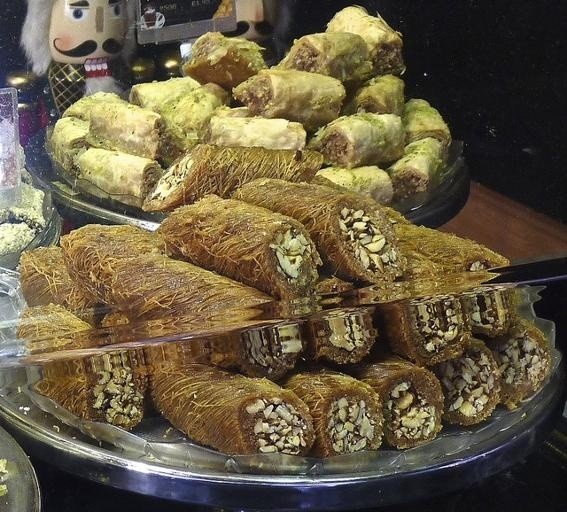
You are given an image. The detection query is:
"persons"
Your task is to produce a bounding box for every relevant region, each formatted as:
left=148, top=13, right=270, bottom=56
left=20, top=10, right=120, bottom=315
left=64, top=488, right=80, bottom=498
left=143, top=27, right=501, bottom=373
left=20, top=1, right=145, bottom=143
